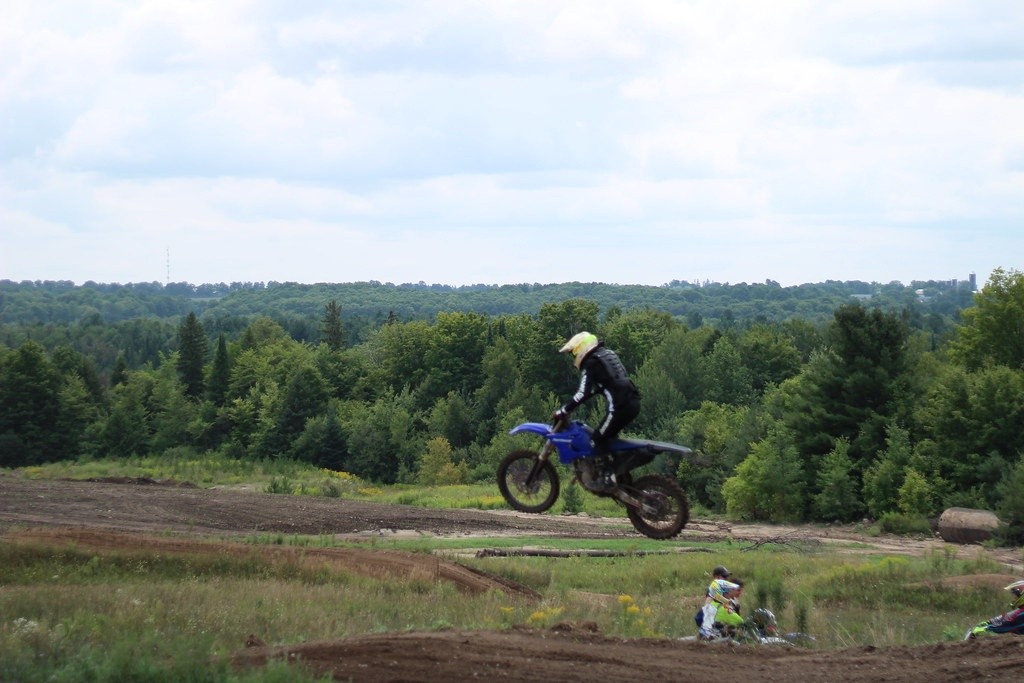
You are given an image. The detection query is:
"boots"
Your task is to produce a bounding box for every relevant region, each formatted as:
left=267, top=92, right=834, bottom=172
left=584, top=453, right=618, bottom=493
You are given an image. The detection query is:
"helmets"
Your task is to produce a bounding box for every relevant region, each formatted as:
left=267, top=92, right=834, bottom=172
left=1004, top=580, right=1024, bottom=610
left=708, top=579, right=740, bottom=604
left=559, top=331, right=598, bottom=370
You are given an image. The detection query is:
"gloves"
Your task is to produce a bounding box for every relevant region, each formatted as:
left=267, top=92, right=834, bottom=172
left=977, top=620, right=989, bottom=626
left=972, top=626, right=988, bottom=637
left=553, top=405, right=570, bottom=421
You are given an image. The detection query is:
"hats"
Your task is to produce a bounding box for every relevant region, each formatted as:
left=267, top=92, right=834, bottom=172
left=714, top=567, right=732, bottom=576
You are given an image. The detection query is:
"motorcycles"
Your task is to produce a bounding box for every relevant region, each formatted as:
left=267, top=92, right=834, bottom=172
left=497, top=400, right=693, bottom=539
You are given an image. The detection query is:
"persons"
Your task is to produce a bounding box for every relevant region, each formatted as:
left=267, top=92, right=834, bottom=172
left=972, top=579, right=1024, bottom=637
left=695, top=566, right=780, bottom=642
left=551, top=330, right=643, bottom=491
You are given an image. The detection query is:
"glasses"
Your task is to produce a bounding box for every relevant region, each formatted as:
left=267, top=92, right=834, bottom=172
left=718, top=575, right=728, bottom=578
